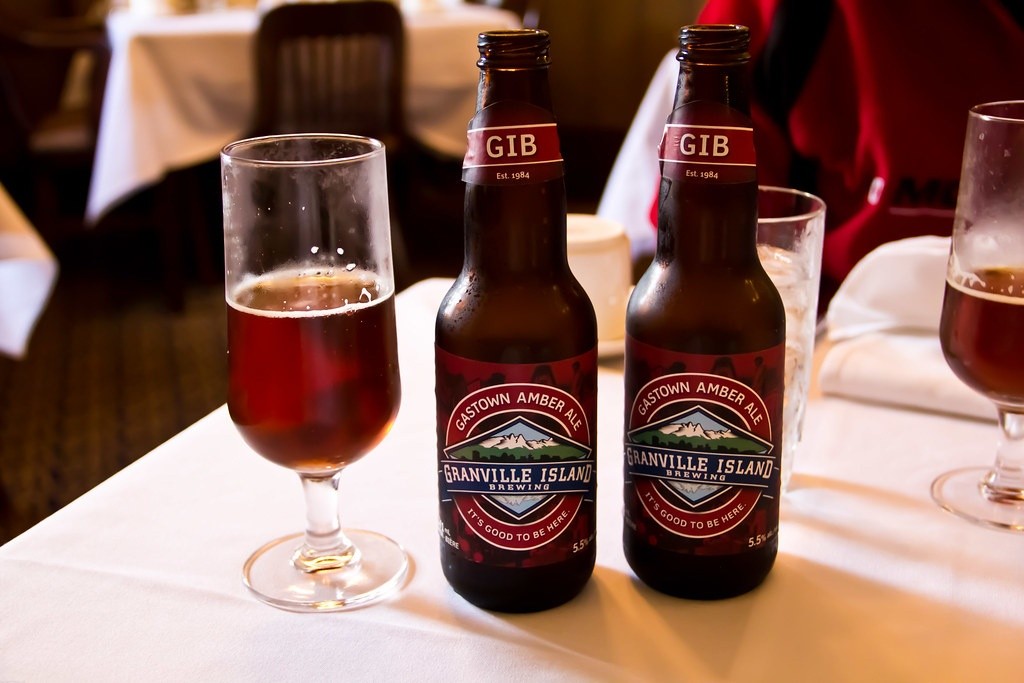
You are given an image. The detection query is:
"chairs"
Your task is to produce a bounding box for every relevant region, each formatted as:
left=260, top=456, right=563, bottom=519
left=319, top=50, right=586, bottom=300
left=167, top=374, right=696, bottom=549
left=9, top=19, right=113, bottom=224
left=249, top=1, right=405, bottom=185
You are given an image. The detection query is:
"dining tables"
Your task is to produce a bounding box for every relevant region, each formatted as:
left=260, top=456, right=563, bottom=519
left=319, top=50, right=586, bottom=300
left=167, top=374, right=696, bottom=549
left=2, top=277, right=1024, bottom=681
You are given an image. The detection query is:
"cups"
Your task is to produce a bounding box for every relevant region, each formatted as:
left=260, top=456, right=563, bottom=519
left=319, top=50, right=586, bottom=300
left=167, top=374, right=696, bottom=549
left=564, top=207, right=629, bottom=356
left=217, top=132, right=413, bottom=611
left=754, top=182, right=829, bottom=468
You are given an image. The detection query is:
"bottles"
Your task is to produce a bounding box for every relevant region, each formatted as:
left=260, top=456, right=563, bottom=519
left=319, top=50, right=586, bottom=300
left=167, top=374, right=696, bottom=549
left=431, top=29, right=601, bottom=615
left=623, top=27, right=788, bottom=604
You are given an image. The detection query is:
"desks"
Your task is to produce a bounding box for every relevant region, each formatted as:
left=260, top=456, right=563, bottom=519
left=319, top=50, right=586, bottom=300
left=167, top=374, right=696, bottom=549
left=104, top=2, right=520, bottom=257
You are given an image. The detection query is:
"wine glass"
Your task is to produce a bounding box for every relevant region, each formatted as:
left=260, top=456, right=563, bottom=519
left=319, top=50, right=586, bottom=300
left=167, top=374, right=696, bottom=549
left=932, top=96, right=1023, bottom=534
